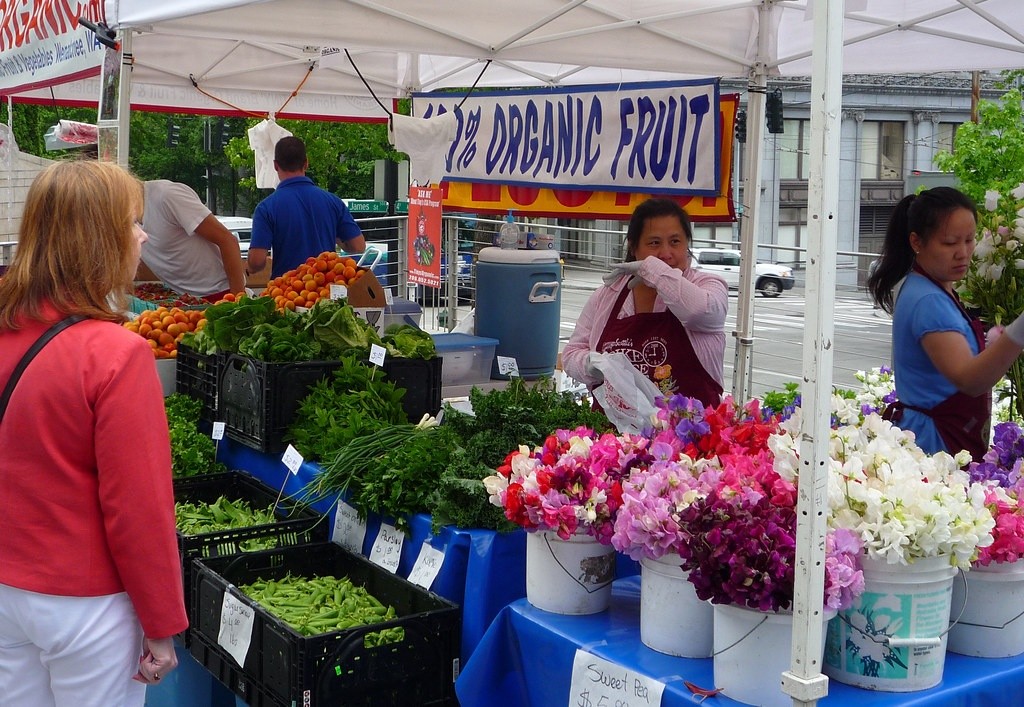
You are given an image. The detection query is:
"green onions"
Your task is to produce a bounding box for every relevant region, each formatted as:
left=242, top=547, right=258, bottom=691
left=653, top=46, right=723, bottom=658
left=279, top=413, right=440, bottom=535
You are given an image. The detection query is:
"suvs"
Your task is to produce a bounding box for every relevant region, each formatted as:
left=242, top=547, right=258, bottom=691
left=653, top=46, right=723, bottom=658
left=686, top=247, right=795, bottom=298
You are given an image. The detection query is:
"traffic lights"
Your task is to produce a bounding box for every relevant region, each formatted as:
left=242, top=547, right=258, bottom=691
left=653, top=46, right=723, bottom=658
left=735, top=113, right=746, bottom=143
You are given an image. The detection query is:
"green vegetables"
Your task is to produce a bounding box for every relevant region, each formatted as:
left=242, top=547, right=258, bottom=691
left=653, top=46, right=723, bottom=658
left=352, top=375, right=617, bottom=530
left=181, top=296, right=436, bottom=373
left=283, top=355, right=407, bottom=460
left=163, top=392, right=228, bottom=478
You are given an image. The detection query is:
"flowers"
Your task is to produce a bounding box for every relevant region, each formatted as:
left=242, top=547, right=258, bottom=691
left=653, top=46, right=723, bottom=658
left=483, top=182, right=1024, bottom=612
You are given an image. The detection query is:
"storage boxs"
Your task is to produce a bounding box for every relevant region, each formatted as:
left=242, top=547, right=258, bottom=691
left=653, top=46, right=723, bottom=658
left=174, top=470, right=461, bottom=707
left=384, top=296, right=423, bottom=335
left=154, top=332, right=498, bottom=453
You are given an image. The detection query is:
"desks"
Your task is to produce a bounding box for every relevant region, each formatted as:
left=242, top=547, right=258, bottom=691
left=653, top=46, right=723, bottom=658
left=454, top=576, right=1024, bottom=707
left=211, top=435, right=641, bottom=674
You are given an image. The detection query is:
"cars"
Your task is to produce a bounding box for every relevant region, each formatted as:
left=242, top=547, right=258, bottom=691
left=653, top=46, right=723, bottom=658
left=214, top=198, right=494, bottom=307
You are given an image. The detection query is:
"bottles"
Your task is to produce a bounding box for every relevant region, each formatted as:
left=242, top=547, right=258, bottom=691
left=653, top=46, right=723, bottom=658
left=500, top=210, right=519, bottom=249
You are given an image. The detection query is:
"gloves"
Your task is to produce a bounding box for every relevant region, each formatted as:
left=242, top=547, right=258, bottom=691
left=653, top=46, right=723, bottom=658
left=585, top=352, right=605, bottom=379
left=1005, top=311, right=1024, bottom=349
left=602, top=260, right=643, bottom=289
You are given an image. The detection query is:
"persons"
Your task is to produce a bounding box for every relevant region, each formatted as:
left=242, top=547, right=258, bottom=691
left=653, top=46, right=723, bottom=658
left=561, top=199, right=730, bottom=435
left=131, top=178, right=245, bottom=304
left=246, top=136, right=366, bottom=285
left=866, top=185, right=1024, bottom=474
left=1, top=162, right=190, bottom=707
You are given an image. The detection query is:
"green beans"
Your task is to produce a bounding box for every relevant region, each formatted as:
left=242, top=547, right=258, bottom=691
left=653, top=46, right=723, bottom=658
left=232, top=572, right=405, bottom=649
left=175, top=493, right=313, bottom=557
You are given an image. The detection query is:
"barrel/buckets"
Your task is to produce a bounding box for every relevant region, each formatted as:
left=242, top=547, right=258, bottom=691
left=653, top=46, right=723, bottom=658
left=713, top=605, right=840, bottom=707
left=819, top=553, right=966, bottom=693
left=640, top=549, right=714, bottom=658
left=947, top=558, right=1024, bottom=658
left=525, top=524, right=614, bottom=616
left=472, top=245, right=561, bottom=380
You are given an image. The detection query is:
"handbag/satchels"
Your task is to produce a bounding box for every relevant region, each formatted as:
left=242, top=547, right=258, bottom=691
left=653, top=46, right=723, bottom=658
left=591, top=349, right=664, bottom=437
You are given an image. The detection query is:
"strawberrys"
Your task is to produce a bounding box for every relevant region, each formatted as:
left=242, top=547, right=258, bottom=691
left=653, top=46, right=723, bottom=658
left=131, top=282, right=203, bottom=308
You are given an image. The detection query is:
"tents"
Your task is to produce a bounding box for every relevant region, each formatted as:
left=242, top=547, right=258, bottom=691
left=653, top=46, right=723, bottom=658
left=0, top=0, right=1024, bottom=705
